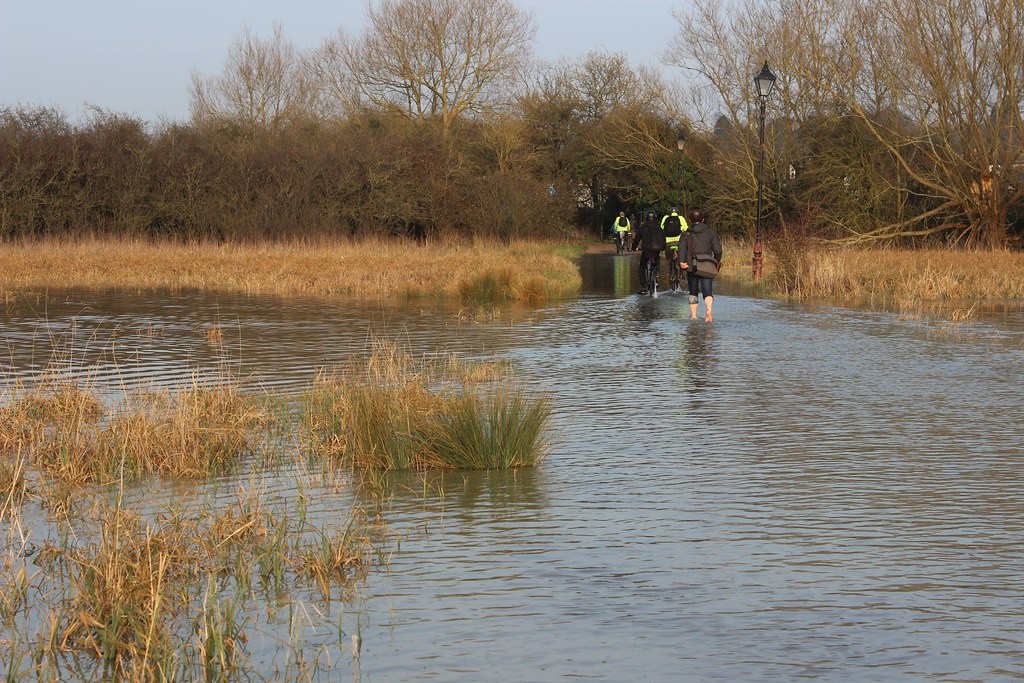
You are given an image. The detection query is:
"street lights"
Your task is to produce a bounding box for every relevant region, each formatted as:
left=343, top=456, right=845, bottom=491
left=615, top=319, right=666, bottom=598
left=750, top=59, right=779, bottom=282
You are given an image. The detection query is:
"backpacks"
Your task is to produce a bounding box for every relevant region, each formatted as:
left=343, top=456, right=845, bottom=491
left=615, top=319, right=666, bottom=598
left=618, top=216, right=628, bottom=227
left=663, top=214, right=682, bottom=237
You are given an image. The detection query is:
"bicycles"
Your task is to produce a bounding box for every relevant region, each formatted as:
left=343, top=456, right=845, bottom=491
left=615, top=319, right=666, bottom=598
left=625, top=232, right=633, bottom=252
left=635, top=247, right=660, bottom=297
left=666, top=245, right=684, bottom=291
left=615, top=232, right=626, bottom=255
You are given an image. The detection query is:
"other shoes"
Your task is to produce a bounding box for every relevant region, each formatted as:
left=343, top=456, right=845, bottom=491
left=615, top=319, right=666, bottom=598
left=653, top=271, right=660, bottom=280
left=637, top=284, right=648, bottom=295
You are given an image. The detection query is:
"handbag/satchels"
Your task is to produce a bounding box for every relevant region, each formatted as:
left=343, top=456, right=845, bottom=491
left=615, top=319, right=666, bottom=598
left=691, top=254, right=719, bottom=279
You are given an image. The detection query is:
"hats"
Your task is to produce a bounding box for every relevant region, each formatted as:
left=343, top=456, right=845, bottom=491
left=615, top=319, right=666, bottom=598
left=644, top=210, right=658, bottom=221
left=668, top=207, right=679, bottom=212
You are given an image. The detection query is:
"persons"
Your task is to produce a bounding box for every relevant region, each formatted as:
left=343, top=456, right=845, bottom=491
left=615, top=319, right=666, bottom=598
left=631, top=210, right=666, bottom=296
left=661, top=207, right=688, bottom=280
left=614, top=211, right=631, bottom=248
left=610, top=221, right=619, bottom=240
left=678, top=212, right=722, bottom=324
